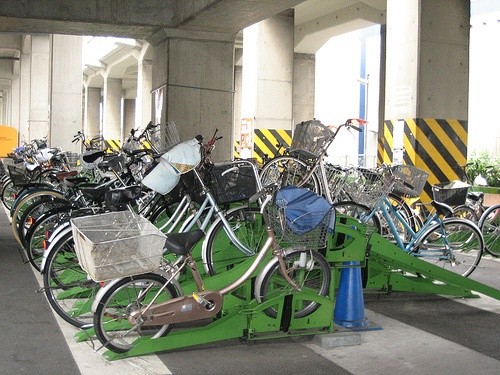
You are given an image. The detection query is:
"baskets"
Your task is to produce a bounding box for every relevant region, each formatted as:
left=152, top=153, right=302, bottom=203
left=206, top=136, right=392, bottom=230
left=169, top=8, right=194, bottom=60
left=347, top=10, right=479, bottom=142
left=205, top=157, right=429, bottom=205
left=1, top=157, right=31, bottom=186
left=289, top=119, right=335, bottom=160
left=148, top=121, right=181, bottom=153
left=432, top=181, right=470, bottom=205
left=70, top=211, right=169, bottom=284
left=268, top=201, right=334, bottom=251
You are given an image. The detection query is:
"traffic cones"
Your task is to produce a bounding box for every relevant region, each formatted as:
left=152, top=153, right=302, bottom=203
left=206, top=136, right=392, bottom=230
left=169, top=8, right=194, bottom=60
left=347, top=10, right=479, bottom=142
left=333, top=224, right=368, bottom=329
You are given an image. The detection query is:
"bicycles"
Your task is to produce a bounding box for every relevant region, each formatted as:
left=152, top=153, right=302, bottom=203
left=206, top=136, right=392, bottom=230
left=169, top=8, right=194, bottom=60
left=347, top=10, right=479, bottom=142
left=69, top=161, right=332, bottom=354
left=257, top=114, right=500, bottom=287
left=0, top=120, right=221, bottom=291
left=36, top=129, right=280, bottom=329
